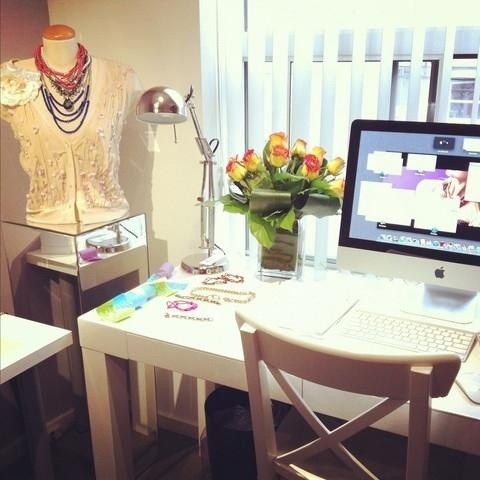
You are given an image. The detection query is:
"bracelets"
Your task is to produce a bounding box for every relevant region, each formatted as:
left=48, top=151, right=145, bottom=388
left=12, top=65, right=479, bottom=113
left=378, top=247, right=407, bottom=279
left=222, top=273, right=243, bottom=284
left=203, top=277, right=225, bottom=285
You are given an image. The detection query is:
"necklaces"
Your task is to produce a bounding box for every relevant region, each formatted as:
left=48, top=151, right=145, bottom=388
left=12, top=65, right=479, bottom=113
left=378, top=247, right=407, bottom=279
left=33, top=41, right=93, bottom=135
left=192, top=287, right=254, bottom=305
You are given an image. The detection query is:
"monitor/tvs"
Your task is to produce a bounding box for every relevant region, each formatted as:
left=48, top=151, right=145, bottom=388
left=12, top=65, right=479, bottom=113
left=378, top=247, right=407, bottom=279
left=336, top=119, right=480, bottom=324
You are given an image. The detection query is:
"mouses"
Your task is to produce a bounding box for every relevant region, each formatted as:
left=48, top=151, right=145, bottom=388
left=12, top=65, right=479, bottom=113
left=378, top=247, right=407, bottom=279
left=455, top=372, right=480, bottom=405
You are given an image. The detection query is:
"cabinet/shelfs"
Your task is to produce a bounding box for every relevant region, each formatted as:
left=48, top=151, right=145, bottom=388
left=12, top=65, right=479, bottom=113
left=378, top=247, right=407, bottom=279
left=1, top=210, right=161, bottom=477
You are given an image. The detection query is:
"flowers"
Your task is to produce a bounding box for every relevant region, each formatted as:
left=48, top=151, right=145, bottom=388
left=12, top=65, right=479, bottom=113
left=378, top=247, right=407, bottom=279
left=216, top=131, right=346, bottom=250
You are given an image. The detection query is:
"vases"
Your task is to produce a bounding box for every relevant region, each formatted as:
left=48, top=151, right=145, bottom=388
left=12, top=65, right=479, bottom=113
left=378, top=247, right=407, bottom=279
left=255, top=218, right=305, bottom=282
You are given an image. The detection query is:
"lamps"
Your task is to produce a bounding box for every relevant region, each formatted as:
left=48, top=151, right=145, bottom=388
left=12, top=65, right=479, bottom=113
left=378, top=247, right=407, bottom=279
left=134, top=87, right=229, bottom=276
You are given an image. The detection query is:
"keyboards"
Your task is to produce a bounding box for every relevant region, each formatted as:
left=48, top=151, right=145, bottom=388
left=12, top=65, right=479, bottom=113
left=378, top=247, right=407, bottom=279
left=339, top=310, right=477, bottom=362
